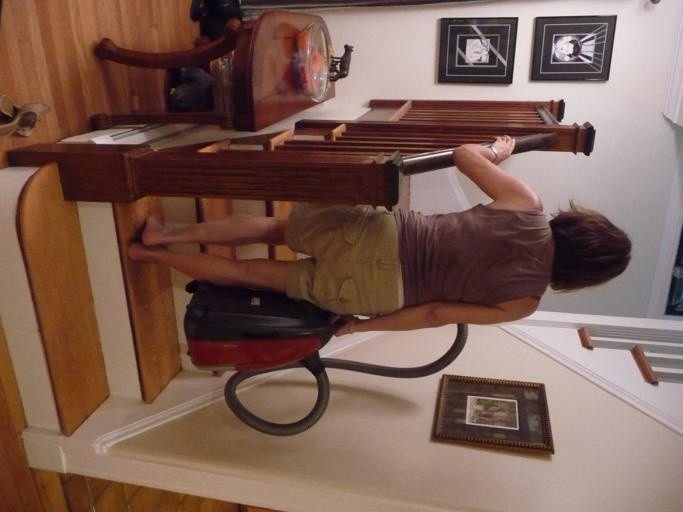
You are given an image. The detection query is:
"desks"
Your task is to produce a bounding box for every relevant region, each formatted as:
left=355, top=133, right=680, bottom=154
left=89, top=9, right=337, bottom=133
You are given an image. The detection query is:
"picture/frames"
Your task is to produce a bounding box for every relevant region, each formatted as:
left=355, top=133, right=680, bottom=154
left=437, top=16, right=519, bottom=85
left=531, top=15, right=618, bottom=81
left=430, top=373, right=555, bottom=455
